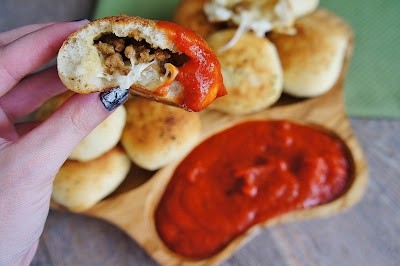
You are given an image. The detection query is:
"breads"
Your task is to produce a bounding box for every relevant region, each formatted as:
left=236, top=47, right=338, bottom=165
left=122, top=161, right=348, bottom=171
left=32, top=91, right=128, bottom=163
left=174, top=0, right=222, bottom=38
left=120, top=99, right=202, bottom=172
left=52, top=146, right=132, bottom=214
left=267, top=17, right=347, bottom=98
left=204, top=29, right=283, bottom=116
left=202, top=0, right=320, bottom=51
left=56, top=13, right=229, bottom=113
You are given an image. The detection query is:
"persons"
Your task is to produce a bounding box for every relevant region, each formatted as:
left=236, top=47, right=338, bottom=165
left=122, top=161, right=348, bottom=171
left=0, top=18, right=130, bottom=266
